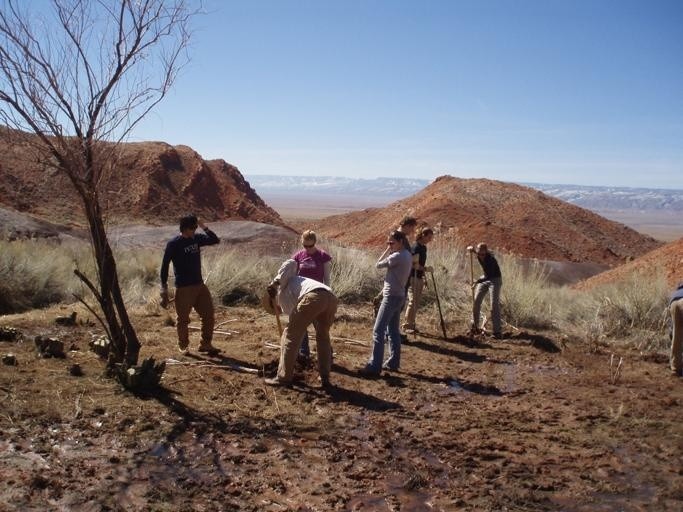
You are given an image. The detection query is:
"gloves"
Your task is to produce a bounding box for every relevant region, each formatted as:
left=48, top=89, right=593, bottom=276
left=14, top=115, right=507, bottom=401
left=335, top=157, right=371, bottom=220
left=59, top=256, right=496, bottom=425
left=160, top=293, right=169, bottom=308
left=373, top=293, right=383, bottom=305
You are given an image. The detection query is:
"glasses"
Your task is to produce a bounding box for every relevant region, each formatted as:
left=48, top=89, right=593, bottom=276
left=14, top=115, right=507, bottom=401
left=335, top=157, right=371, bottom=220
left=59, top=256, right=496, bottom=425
left=302, top=244, right=315, bottom=247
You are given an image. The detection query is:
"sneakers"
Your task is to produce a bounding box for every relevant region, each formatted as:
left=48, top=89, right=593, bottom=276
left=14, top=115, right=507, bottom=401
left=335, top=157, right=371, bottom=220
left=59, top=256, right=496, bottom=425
left=265, top=376, right=292, bottom=386
left=402, top=326, right=418, bottom=333
left=317, top=376, right=330, bottom=384
left=178, top=346, right=190, bottom=355
left=198, top=345, right=222, bottom=352
left=360, top=367, right=380, bottom=379
left=381, top=361, right=395, bottom=372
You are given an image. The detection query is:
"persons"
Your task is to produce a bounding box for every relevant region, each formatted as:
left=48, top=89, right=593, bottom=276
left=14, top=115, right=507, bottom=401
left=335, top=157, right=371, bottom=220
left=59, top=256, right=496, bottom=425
left=401, top=228, right=435, bottom=334
left=160, top=215, right=221, bottom=356
left=466, top=242, right=502, bottom=339
left=670, top=285, right=683, bottom=374
left=386, top=217, right=417, bottom=340
left=358, top=230, right=413, bottom=375
left=263, top=258, right=338, bottom=389
left=290, top=228, right=332, bottom=357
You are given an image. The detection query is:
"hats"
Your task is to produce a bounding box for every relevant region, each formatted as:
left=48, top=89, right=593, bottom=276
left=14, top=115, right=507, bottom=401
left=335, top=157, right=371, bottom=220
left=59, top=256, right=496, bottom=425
left=263, top=292, right=282, bottom=315
